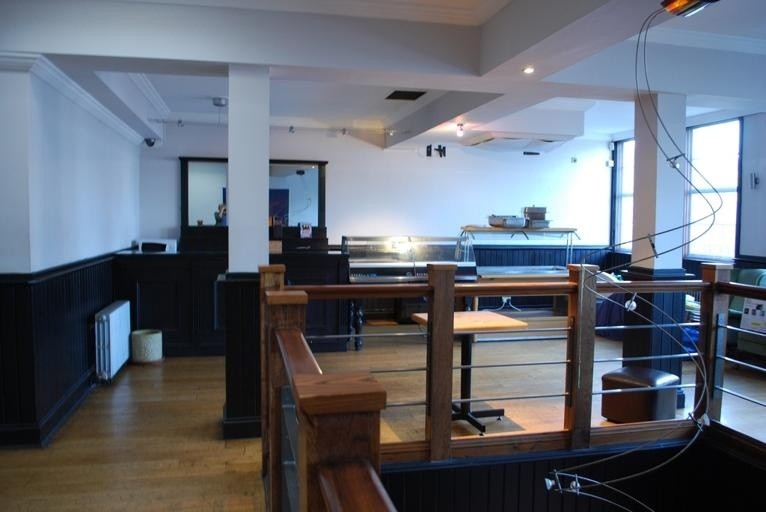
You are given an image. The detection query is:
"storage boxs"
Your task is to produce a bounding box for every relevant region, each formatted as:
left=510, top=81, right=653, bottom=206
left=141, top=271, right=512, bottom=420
left=525, top=208, right=551, bottom=230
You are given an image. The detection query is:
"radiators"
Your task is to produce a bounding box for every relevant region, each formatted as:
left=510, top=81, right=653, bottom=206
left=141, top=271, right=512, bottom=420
left=92, top=298, right=131, bottom=382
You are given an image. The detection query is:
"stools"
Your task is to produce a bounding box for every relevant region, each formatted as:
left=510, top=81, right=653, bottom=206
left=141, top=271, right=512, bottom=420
left=600, top=365, right=679, bottom=422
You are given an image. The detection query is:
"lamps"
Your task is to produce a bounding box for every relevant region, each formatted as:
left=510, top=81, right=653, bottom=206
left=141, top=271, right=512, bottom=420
left=456, top=123, right=464, bottom=138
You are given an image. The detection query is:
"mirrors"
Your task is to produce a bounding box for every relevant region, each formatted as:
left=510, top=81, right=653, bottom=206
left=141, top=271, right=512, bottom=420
left=178, top=156, right=329, bottom=238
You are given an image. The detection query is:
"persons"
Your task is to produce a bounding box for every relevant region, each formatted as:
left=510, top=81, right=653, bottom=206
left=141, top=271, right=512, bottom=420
left=213, top=203, right=227, bottom=227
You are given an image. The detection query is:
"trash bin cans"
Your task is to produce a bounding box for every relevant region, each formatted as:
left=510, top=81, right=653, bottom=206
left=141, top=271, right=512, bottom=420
left=131, top=328, right=163, bottom=365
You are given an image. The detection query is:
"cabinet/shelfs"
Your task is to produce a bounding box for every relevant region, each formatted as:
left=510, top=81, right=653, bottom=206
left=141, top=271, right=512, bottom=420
left=341, top=234, right=478, bottom=318
left=461, top=226, right=578, bottom=313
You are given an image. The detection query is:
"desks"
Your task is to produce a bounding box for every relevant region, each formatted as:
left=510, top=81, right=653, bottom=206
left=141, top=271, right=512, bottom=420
left=411, top=309, right=529, bottom=436
